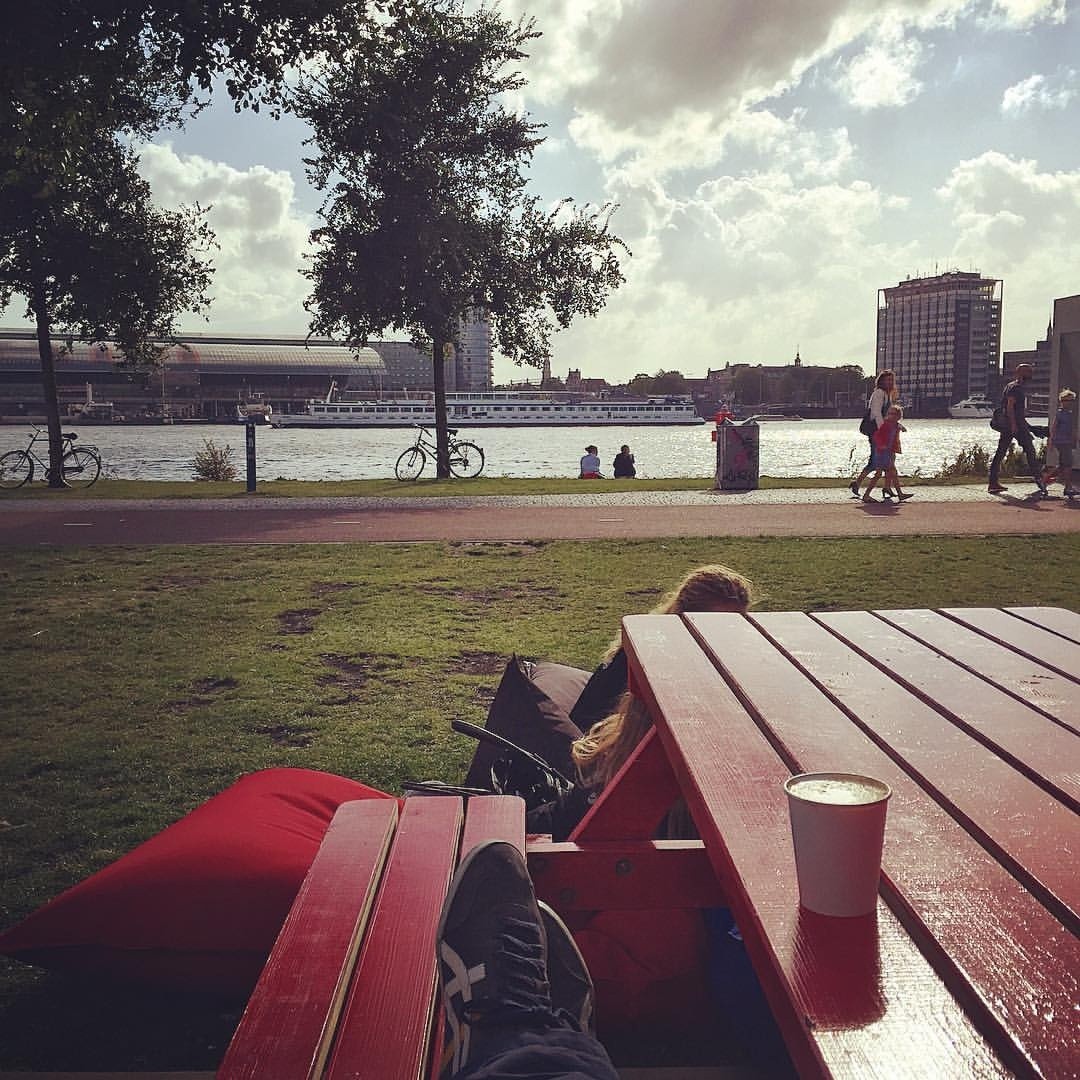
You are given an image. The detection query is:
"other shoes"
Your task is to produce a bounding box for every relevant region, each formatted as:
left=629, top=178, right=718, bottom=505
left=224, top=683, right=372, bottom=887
left=863, top=494, right=877, bottom=503
left=1035, top=478, right=1048, bottom=495
left=1064, top=488, right=1080, bottom=495
left=987, top=481, right=1008, bottom=493
left=899, top=494, right=914, bottom=500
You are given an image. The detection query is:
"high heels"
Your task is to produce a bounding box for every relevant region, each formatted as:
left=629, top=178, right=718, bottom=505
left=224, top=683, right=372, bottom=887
left=848, top=480, right=860, bottom=496
left=882, top=488, right=898, bottom=498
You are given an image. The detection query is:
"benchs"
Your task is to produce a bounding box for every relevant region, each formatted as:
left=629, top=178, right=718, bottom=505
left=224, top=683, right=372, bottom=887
left=211, top=795, right=528, bottom=1080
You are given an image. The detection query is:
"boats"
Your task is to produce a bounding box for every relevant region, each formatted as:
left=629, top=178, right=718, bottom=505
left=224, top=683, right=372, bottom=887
left=753, top=414, right=803, bottom=421
left=270, top=375, right=706, bottom=428
left=952, top=393, right=994, bottom=418
left=237, top=384, right=272, bottom=425
left=68, top=382, right=173, bottom=426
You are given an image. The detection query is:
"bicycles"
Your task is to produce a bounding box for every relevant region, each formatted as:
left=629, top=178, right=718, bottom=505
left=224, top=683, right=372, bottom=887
left=0, top=423, right=102, bottom=489
left=395, top=426, right=484, bottom=481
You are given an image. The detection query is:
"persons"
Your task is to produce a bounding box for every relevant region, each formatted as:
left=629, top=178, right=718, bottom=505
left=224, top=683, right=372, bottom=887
left=613, top=445, right=636, bottom=479
left=525, top=565, right=751, bottom=843
left=433, top=840, right=619, bottom=1080
left=581, top=446, right=600, bottom=479
left=862, top=405, right=913, bottom=502
left=1037, top=390, right=1080, bottom=495
left=848, top=370, right=907, bottom=498
left=988, top=363, right=1042, bottom=490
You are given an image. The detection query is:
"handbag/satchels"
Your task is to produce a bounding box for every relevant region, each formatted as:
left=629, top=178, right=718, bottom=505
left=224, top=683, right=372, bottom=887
left=1046, top=447, right=1059, bottom=465
left=859, top=415, right=876, bottom=437
left=990, top=407, right=1010, bottom=432
left=872, top=446, right=891, bottom=465
left=399, top=719, right=587, bottom=843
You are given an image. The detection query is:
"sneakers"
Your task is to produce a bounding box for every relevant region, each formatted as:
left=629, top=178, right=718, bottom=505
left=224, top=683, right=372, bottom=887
left=536, top=899, right=592, bottom=1030
left=435, top=839, right=551, bottom=1080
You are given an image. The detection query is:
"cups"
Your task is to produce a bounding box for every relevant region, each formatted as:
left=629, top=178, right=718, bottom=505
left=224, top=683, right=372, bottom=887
left=784, top=772, right=893, bottom=917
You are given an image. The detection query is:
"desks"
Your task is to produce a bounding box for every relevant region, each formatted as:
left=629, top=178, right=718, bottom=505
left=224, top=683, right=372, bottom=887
left=567, top=604, right=1080, bottom=1080
